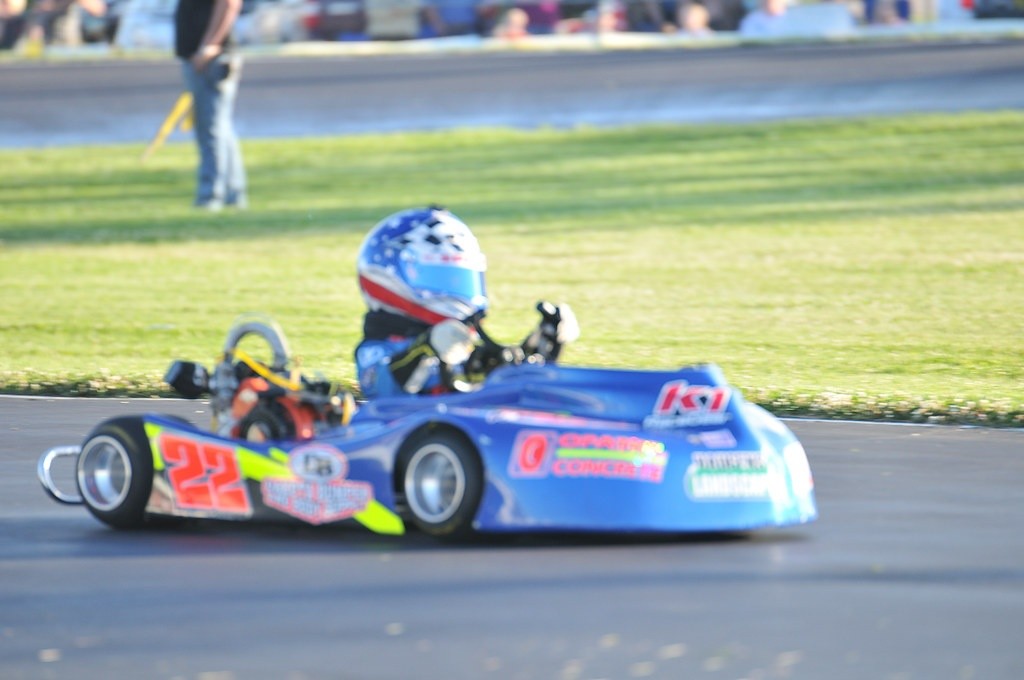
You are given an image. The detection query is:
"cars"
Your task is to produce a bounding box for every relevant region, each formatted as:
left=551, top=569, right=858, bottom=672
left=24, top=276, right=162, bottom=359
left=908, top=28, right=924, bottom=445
left=108, top=0, right=331, bottom=57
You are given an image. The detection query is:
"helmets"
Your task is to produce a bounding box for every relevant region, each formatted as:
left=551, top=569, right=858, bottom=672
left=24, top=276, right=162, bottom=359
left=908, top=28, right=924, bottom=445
left=357, top=207, right=489, bottom=328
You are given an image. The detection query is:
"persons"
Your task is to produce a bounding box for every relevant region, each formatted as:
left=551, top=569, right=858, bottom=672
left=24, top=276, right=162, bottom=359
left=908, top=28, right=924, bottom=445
left=174, top=0, right=247, bottom=209
left=0, top=0, right=121, bottom=53
left=410, top=0, right=1024, bottom=41
left=355, top=205, right=578, bottom=394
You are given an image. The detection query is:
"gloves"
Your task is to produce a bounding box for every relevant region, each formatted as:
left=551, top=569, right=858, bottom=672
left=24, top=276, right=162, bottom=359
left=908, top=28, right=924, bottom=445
left=539, top=302, right=583, bottom=345
left=429, top=317, right=482, bottom=367
